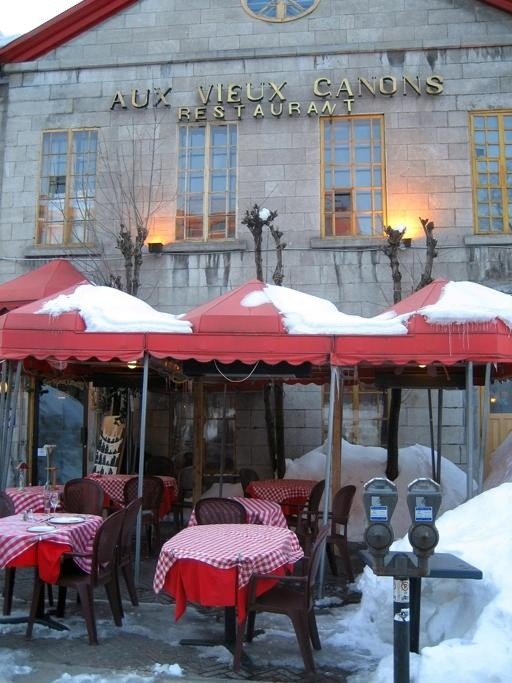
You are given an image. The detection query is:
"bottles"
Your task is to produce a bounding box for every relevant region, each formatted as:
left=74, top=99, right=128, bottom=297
left=22, top=509, right=29, bottom=522
left=28, top=508, right=34, bottom=520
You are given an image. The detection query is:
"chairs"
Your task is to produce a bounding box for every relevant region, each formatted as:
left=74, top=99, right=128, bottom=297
left=240, top=468, right=259, bottom=498
left=195, top=498, right=246, bottom=524
left=0, top=456, right=193, bottom=646
left=296, top=485, right=356, bottom=583
left=280, top=479, right=325, bottom=535
left=246, top=523, right=330, bottom=674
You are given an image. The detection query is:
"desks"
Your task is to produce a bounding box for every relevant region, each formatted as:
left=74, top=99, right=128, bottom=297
left=359, top=551, right=483, bottom=655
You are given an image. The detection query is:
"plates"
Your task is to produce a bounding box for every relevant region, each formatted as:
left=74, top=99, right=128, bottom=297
left=49, top=516, right=87, bottom=525
left=26, top=525, right=56, bottom=533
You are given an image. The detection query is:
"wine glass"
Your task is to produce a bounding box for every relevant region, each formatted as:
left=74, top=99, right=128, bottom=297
left=49, top=489, right=59, bottom=517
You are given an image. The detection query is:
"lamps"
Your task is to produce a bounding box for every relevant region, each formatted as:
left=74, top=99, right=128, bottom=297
left=399, top=239, right=412, bottom=247
left=148, top=243, right=163, bottom=253
